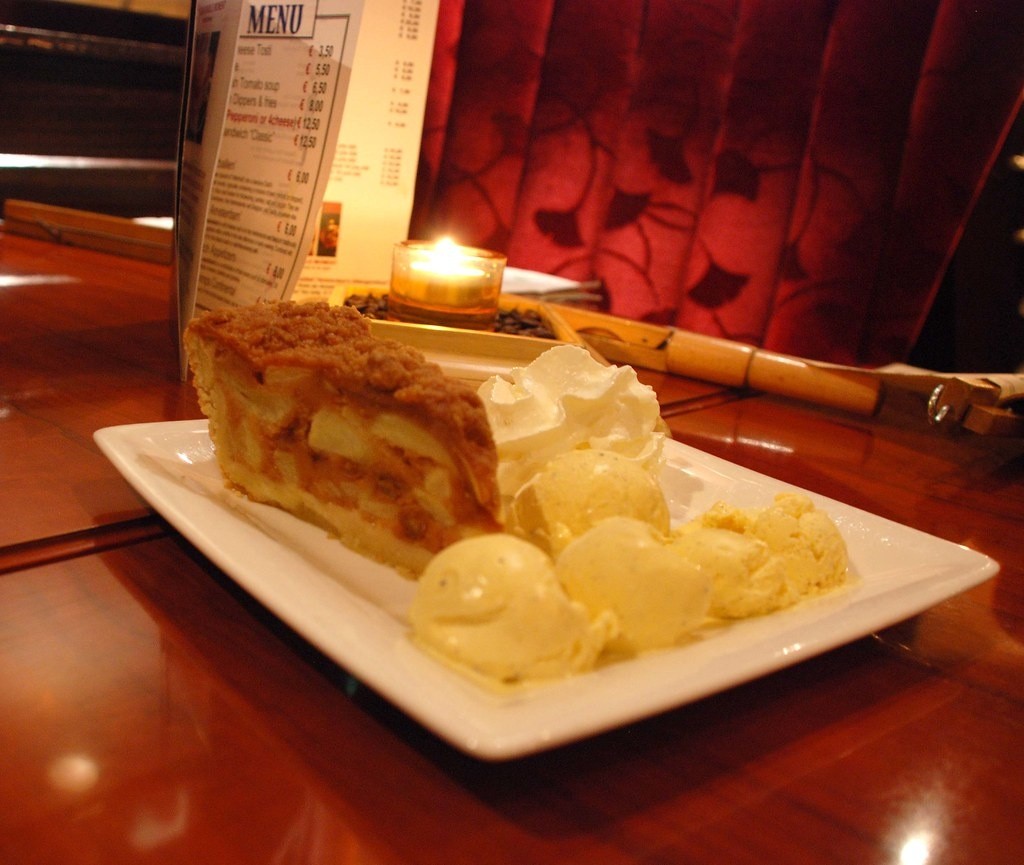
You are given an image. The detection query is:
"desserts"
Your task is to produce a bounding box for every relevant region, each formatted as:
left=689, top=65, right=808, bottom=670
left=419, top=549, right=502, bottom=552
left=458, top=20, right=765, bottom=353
left=186, top=295, right=847, bottom=689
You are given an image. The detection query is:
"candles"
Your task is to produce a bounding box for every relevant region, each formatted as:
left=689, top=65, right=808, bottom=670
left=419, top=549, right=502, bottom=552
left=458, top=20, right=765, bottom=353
left=386, top=239, right=508, bottom=330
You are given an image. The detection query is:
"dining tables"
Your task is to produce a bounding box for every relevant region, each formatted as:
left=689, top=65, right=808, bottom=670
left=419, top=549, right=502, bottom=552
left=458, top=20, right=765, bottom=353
left=1, top=388, right=1024, bottom=865
left=0, top=230, right=735, bottom=554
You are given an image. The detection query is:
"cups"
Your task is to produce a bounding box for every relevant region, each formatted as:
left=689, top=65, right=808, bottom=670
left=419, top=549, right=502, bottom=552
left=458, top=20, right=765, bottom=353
left=387, top=238, right=508, bottom=332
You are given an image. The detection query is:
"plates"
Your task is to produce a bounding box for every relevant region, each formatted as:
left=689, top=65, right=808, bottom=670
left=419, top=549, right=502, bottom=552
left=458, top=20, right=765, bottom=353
left=92, top=419, right=1000, bottom=764
